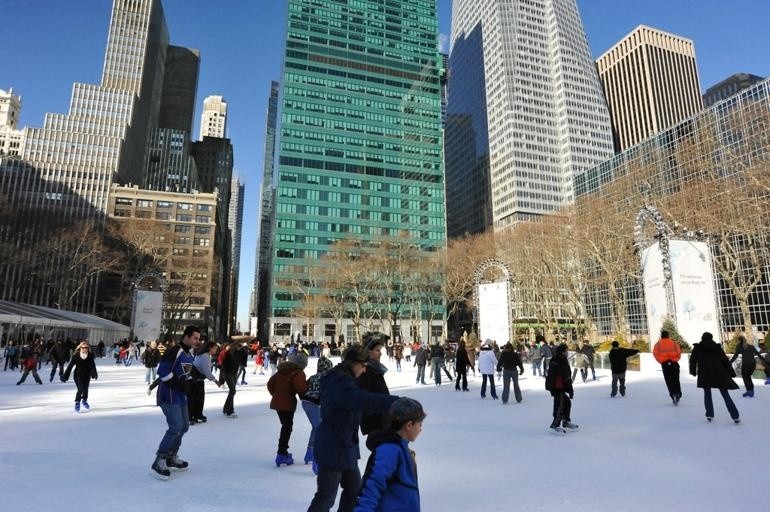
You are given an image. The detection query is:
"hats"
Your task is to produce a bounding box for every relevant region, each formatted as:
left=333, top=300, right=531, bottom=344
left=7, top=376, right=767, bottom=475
left=504, top=341, right=513, bottom=348
left=288, top=350, right=308, bottom=368
left=485, top=338, right=493, bottom=345
left=391, top=396, right=427, bottom=424
left=342, top=344, right=371, bottom=363
left=362, top=333, right=385, bottom=350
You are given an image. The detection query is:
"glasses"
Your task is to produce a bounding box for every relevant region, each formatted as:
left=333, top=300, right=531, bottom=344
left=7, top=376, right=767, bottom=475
left=237, top=345, right=242, bottom=347
left=80, top=347, right=89, bottom=349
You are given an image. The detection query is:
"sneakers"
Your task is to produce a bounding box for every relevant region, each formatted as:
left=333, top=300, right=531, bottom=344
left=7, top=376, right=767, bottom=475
left=733, top=417, right=740, bottom=423
left=517, top=398, right=522, bottom=402
left=742, top=391, right=753, bottom=397
left=227, top=412, right=237, bottom=416
left=16, top=381, right=23, bottom=385
left=550, top=425, right=566, bottom=433
left=60, top=375, right=66, bottom=383
left=194, top=415, right=207, bottom=420
left=705, top=416, right=712, bottom=420
left=492, top=395, right=499, bottom=399
left=593, top=375, right=595, bottom=379
left=621, top=392, right=625, bottom=397
left=38, top=380, right=42, bottom=384
left=583, top=379, right=586, bottom=382
left=455, top=386, right=461, bottom=389
left=503, top=400, right=508, bottom=402
left=276, top=454, right=293, bottom=464
left=304, top=449, right=315, bottom=461
left=481, top=394, right=486, bottom=397
left=421, top=382, right=426, bottom=385
left=50, top=377, right=54, bottom=382
left=241, top=381, right=247, bottom=384
left=416, top=378, right=419, bottom=384
left=462, top=388, right=469, bottom=391
left=765, top=378, right=770, bottom=384
left=82, top=400, right=90, bottom=409
left=189, top=416, right=196, bottom=422
left=562, top=421, right=579, bottom=429
left=146, top=385, right=152, bottom=395
left=312, top=461, right=318, bottom=471
left=166, top=456, right=188, bottom=468
left=75, top=402, right=80, bottom=411
left=152, top=459, right=170, bottom=476
left=610, top=393, right=616, bottom=398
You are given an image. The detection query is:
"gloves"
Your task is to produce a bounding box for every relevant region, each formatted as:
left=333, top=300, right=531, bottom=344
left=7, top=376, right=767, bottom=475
left=180, top=382, right=192, bottom=396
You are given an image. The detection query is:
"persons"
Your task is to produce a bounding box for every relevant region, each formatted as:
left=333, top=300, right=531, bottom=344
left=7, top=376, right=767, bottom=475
left=357, top=336, right=392, bottom=497
left=384, top=334, right=556, bottom=378
left=579, top=340, right=596, bottom=381
left=609, top=340, right=642, bottom=397
left=690, top=332, right=743, bottom=425
left=300, top=354, right=332, bottom=473
left=187, top=342, right=220, bottom=426
left=268, top=351, right=309, bottom=465
left=142, top=341, right=160, bottom=386
left=728, top=335, right=769, bottom=398
left=497, top=342, right=525, bottom=404
left=413, top=348, right=430, bottom=385
left=546, top=343, right=580, bottom=436
left=454, top=341, right=476, bottom=391
left=570, top=348, right=591, bottom=383
left=653, top=329, right=682, bottom=405
left=149, top=325, right=201, bottom=480
left=3, top=336, right=75, bottom=385
left=307, top=343, right=398, bottom=512
left=219, top=340, right=243, bottom=418
left=756, top=343, right=770, bottom=385
left=157, top=342, right=166, bottom=357
left=350, top=396, right=427, bottom=512
left=203, top=336, right=379, bottom=387
left=96, top=336, right=149, bottom=367
left=63, top=340, right=100, bottom=413
